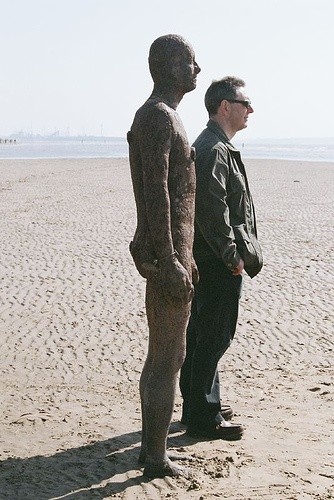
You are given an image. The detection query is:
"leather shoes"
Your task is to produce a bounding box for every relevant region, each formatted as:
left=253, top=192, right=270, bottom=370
left=181, top=405, right=243, bottom=438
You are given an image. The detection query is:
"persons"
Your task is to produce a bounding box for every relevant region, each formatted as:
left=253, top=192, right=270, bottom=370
left=129, top=35, right=201, bottom=478
left=180, top=77, right=264, bottom=442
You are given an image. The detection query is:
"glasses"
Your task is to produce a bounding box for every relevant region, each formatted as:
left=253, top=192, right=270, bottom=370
left=222, top=97, right=254, bottom=111
